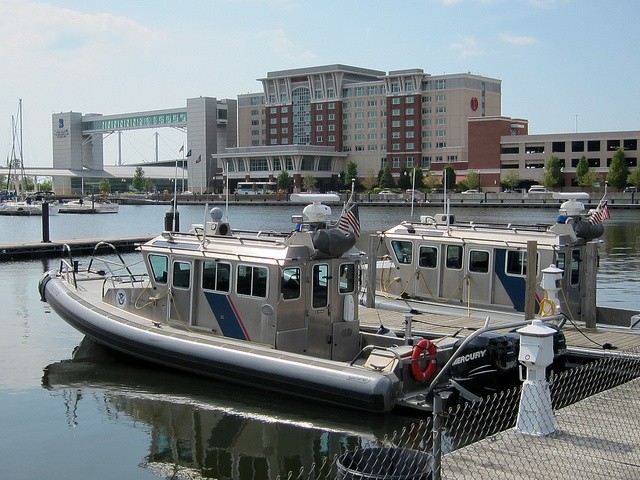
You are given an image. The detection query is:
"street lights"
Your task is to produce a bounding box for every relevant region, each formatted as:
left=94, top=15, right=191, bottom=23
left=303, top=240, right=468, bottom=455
left=404, top=171, right=408, bottom=192
left=560, top=167, right=564, bottom=192
left=477, top=169, right=481, bottom=192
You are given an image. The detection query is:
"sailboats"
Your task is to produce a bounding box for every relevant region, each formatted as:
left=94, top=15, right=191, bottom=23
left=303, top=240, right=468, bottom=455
left=0, top=98, right=58, bottom=216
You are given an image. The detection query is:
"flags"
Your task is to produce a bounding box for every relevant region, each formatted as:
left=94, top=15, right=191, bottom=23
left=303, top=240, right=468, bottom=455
left=186, top=149, right=191, bottom=157
left=195, top=154, right=201, bottom=164
left=589, top=202, right=611, bottom=227
left=338, top=203, right=361, bottom=238
left=179, top=145, right=183, bottom=153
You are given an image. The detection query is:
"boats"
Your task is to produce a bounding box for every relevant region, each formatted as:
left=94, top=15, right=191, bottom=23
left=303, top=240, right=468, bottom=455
left=58, top=193, right=120, bottom=214
left=38, top=158, right=566, bottom=417
left=286, top=169, right=640, bottom=375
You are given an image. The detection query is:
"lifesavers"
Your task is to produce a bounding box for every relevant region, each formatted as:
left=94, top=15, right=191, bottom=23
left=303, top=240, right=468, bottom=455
left=411, top=340, right=437, bottom=382
